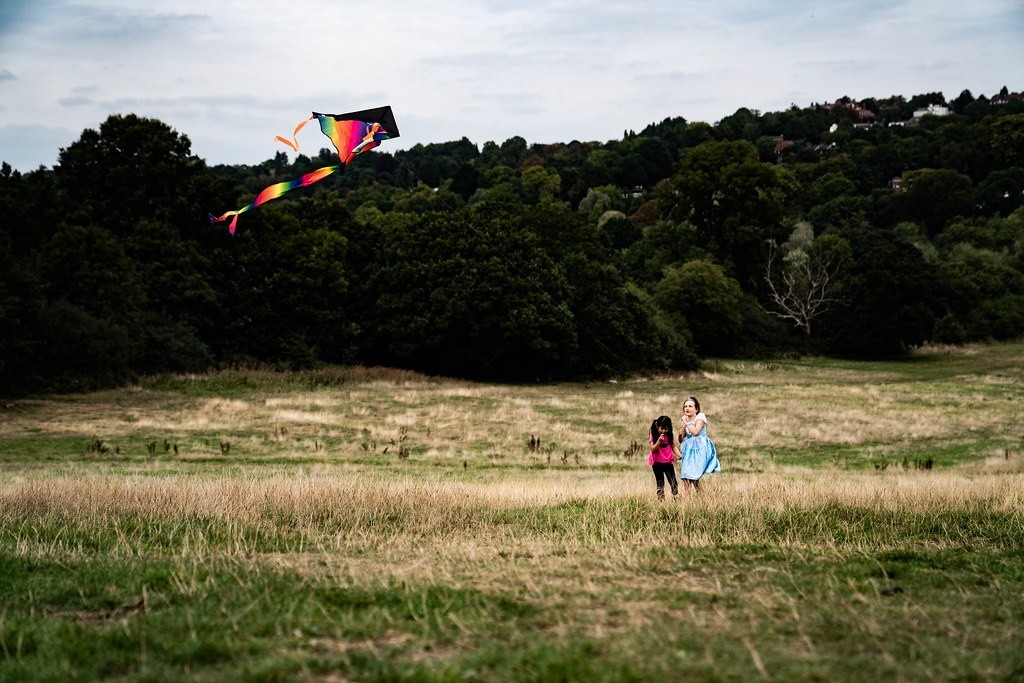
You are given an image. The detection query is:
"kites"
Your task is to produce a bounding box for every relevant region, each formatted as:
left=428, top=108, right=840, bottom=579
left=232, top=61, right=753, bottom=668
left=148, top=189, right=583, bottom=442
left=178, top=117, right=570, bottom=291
left=208, top=106, right=400, bottom=235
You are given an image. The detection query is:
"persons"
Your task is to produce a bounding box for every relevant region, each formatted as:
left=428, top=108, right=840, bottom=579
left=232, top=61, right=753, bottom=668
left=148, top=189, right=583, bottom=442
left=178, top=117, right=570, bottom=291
left=648, top=416, right=682, bottom=502
left=678, top=397, right=721, bottom=492
